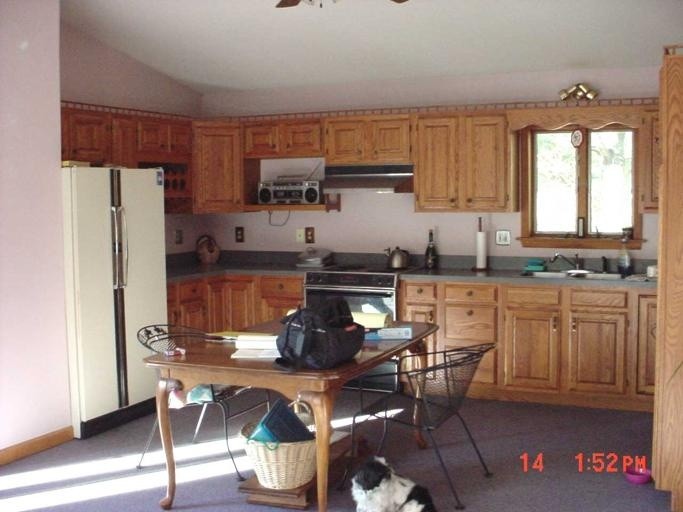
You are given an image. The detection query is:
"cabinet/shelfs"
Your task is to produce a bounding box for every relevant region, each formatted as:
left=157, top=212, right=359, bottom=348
left=242, top=113, right=325, bottom=159
left=397, top=270, right=438, bottom=396
left=193, top=119, right=243, bottom=214
left=138, top=110, right=194, bottom=164
left=437, top=274, right=500, bottom=401
left=630, top=273, right=656, bottom=413
left=501, top=267, right=630, bottom=412
left=638, top=109, right=661, bottom=213
left=166, top=268, right=205, bottom=322
left=208, top=264, right=255, bottom=326
left=652, top=46, right=683, bottom=510
left=258, top=267, right=304, bottom=323
left=323, top=107, right=411, bottom=166
left=411, top=107, right=513, bottom=214
left=62, top=101, right=140, bottom=166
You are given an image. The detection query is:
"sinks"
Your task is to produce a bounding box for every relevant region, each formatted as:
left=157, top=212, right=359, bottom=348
left=572, top=272, right=622, bottom=282
left=523, top=271, right=570, bottom=280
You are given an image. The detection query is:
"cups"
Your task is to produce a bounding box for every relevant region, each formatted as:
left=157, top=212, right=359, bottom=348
left=646, top=266, right=658, bottom=277
left=622, top=228, right=633, bottom=240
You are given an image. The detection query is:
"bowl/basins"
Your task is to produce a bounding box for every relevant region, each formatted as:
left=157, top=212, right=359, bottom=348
left=624, top=468, right=650, bottom=484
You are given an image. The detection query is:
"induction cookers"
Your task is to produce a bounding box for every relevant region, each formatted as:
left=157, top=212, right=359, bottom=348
left=306, top=263, right=426, bottom=273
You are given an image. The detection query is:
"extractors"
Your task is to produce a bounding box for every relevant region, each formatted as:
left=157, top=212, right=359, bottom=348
left=324, top=164, right=414, bottom=189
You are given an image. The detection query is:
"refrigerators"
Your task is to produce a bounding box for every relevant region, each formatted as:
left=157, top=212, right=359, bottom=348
left=60, top=165, right=169, bottom=441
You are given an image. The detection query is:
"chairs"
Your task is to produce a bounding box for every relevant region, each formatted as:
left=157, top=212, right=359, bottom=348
left=335, top=343, right=496, bottom=510
left=138, top=324, right=270, bottom=481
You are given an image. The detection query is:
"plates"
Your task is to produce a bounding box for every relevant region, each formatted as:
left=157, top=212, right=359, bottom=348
left=532, top=271, right=569, bottom=278
left=585, top=272, right=621, bottom=279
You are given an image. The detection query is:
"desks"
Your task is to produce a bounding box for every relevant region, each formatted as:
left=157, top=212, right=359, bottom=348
left=144, top=321, right=437, bottom=511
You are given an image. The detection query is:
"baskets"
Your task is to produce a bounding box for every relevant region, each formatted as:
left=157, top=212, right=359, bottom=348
left=238, top=419, right=335, bottom=490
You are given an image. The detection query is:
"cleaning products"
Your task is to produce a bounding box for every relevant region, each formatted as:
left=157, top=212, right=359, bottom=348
left=615, top=237, right=632, bottom=275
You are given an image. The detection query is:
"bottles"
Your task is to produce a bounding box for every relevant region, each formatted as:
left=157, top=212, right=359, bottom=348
left=617, top=239, right=630, bottom=277
left=425, top=229, right=438, bottom=269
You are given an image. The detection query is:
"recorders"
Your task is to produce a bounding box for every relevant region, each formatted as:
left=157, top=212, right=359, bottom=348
left=257, top=180, right=323, bottom=204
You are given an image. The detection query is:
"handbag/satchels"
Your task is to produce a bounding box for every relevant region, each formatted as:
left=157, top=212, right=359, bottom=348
left=276, top=297, right=366, bottom=369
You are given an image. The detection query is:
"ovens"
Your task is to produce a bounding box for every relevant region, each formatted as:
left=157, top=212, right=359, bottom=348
left=303, top=285, right=401, bottom=393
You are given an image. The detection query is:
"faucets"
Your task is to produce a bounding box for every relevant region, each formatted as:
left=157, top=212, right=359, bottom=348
left=548, top=253, right=583, bottom=268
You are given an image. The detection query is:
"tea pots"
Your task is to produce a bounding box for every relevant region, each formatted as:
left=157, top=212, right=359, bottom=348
left=383, top=246, right=412, bottom=269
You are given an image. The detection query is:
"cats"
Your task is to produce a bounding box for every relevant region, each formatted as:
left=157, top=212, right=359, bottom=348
left=350, top=455, right=437, bottom=512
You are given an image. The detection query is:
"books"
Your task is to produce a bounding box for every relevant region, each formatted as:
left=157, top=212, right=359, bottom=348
left=204, top=329, right=283, bottom=361
